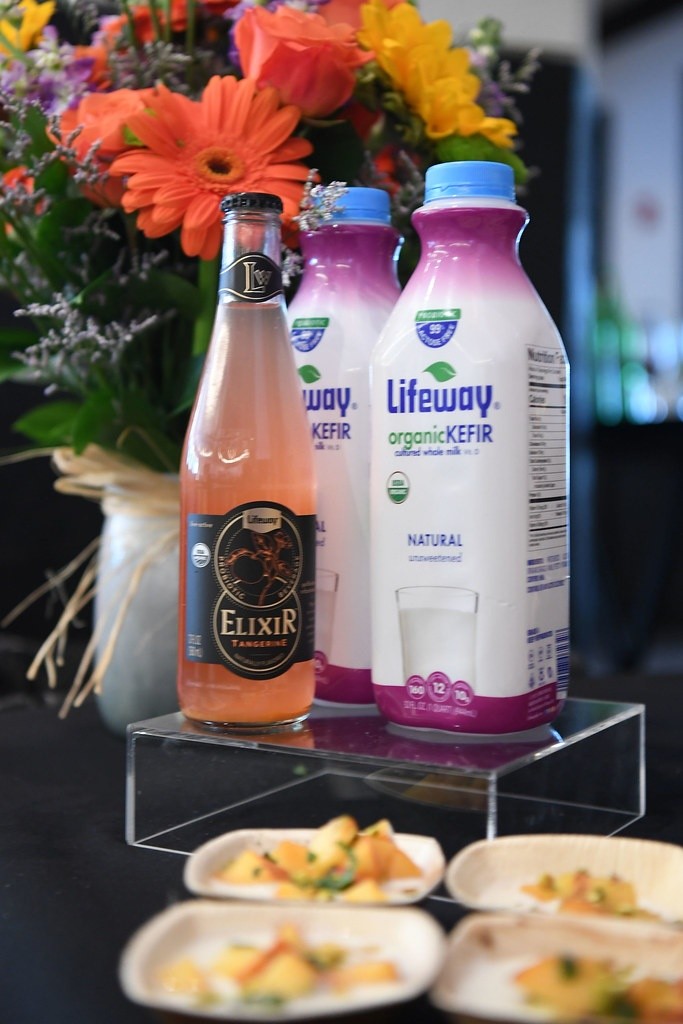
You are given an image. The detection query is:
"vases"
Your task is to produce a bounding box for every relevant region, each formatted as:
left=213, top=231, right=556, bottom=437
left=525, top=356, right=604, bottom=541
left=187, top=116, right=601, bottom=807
left=93, top=483, right=180, bottom=741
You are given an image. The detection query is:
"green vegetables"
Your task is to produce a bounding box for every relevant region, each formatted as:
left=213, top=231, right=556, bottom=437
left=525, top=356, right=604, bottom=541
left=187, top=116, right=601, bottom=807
left=242, top=840, right=639, bottom=1020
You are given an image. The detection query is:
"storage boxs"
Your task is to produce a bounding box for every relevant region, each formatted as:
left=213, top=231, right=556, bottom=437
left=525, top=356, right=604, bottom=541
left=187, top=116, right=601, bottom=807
left=126, top=696, right=646, bottom=904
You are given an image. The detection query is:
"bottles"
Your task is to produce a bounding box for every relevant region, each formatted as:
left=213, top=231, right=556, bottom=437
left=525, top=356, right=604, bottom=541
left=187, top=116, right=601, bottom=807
left=368, top=159, right=575, bottom=731
left=176, top=192, right=319, bottom=726
left=279, top=188, right=404, bottom=706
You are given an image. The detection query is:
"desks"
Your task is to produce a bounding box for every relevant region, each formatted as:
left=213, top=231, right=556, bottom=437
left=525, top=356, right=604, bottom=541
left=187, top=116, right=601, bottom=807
left=0, top=671, right=682, bottom=1024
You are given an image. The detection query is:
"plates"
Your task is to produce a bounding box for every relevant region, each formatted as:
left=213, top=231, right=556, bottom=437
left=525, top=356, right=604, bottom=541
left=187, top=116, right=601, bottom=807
left=124, top=898, right=446, bottom=1021
left=425, top=914, right=683, bottom=1023
left=445, top=836, right=683, bottom=926
left=184, top=821, right=448, bottom=905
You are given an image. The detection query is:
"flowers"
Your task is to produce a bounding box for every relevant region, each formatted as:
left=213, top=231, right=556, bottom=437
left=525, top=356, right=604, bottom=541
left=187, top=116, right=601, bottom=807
left=0, top=0, right=540, bottom=476
